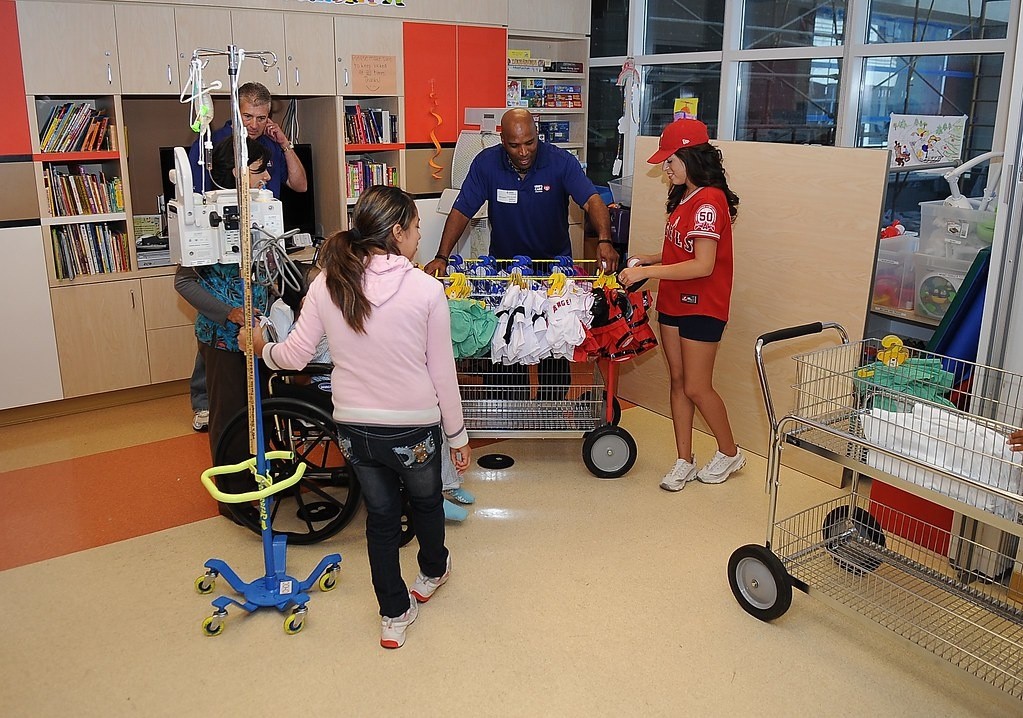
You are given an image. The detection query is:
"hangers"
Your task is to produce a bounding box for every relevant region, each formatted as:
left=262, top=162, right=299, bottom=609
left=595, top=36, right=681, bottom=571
left=858, top=334, right=910, bottom=377
left=443, top=254, right=625, bottom=308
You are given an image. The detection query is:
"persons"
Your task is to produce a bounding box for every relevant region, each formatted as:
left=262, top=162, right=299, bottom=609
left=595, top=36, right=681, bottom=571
left=189, top=83, right=307, bottom=431
left=425, top=108, right=618, bottom=399
left=618, top=118, right=745, bottom=491
left=277, top=259, right=474, bottom=521
left=238, top=186, right=471, bottom=649
left=174, top=137, right=271, bottom=526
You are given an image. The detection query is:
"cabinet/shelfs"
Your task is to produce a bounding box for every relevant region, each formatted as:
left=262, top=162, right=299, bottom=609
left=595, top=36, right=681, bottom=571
left=507, top=27, right=590, bottom=274
left=403, top=21, right=507, bottom=144
left=17, top=0, right=405, bottom=400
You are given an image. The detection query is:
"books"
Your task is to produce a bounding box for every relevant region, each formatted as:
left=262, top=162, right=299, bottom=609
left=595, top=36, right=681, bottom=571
left=346, top=153, right=397, bottom=198
left=40, top=102, right=118, bottom=152
left=43, top=162, right=125, bottom=217
left=344, top=105, right=399, bottom=144
left=51, top=223, right=130, bottom=280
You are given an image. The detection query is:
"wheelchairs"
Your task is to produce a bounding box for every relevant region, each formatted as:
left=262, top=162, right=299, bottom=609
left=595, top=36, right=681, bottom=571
left=208, top=288, right=416, bottom=551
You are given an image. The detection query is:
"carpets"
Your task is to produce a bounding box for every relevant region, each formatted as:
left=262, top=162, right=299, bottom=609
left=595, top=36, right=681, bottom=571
left=0, top=367, right=638, bottom=572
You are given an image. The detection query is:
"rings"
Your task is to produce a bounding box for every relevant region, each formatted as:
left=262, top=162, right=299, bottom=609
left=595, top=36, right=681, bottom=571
left=270, top=126, right=272, bottom=129
left=621, top=277, right=624, bottom=280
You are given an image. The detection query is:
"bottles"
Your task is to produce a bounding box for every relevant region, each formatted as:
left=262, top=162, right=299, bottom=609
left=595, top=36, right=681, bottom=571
left=629, top=259, right=642, bottom=268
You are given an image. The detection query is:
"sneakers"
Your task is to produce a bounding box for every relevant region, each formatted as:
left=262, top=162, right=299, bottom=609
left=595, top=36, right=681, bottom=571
left=192, top=409, right=209, bottom=432
left=659, top=451, right=698, bottom=492
left=696, top=442, right=748, bottom=484
left=408, top=552, right=452, bottom=603
left=380, top=593, right=419, bottom=649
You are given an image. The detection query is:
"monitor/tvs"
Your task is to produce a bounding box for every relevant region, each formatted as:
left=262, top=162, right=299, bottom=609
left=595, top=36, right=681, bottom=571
left=159, top=142, right=315, bottom=257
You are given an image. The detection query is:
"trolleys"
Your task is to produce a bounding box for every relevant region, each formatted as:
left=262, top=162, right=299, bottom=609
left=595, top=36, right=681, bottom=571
left=436, top=252, right=639, bottom=480
left=725, top=319, right=1023, bottom=701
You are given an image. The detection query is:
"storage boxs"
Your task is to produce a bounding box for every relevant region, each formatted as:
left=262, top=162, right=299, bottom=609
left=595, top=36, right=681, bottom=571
left=507, top=49, right=585, bottom=142
left=870, top=196, right=997, bottom=319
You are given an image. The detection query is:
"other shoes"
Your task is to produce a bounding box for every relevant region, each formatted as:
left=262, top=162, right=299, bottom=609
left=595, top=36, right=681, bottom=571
left=217, top=498, right=262, bottom=527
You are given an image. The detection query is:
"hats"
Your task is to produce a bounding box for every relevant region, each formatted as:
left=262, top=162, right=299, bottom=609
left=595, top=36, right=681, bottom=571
left=647, top=117, right=709, bottom=164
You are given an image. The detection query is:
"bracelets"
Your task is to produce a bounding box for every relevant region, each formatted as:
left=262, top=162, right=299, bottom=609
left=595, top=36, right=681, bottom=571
left=283, top=147, right=291, bottom=151
left=598, top=239, right=612, bottom=245
left=435, top=255, right=448, bottom=264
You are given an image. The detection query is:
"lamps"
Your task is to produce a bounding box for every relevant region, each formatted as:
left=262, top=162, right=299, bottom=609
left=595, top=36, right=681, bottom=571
left=943, top=152, right=1004, bottom=210
